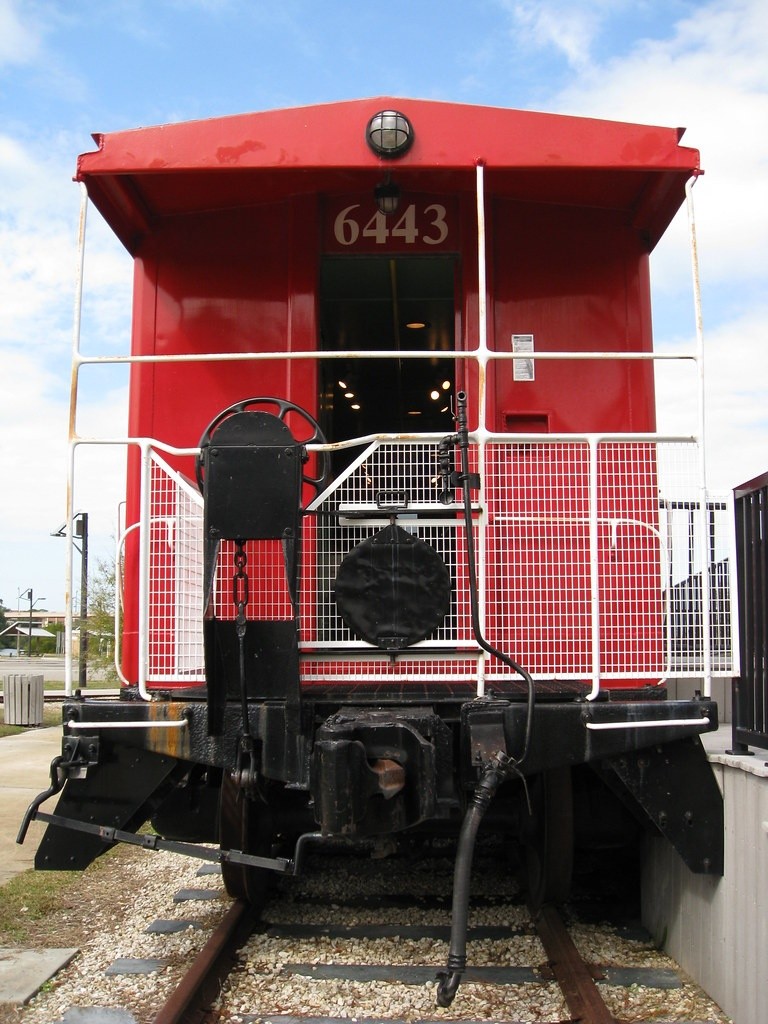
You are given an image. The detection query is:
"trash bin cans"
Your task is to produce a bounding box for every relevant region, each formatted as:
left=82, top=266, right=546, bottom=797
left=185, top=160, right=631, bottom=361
left=3, top=673, right=44, bottom=728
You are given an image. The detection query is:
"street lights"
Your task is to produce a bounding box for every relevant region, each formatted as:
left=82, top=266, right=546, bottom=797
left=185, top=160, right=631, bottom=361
left=50, top=533, right=89, bottom=687
left=28, top=597, right=46, bottom=656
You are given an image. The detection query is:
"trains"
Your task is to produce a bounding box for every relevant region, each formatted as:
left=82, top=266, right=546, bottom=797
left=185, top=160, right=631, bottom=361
left=18, top=96, right=735, bottom=930
